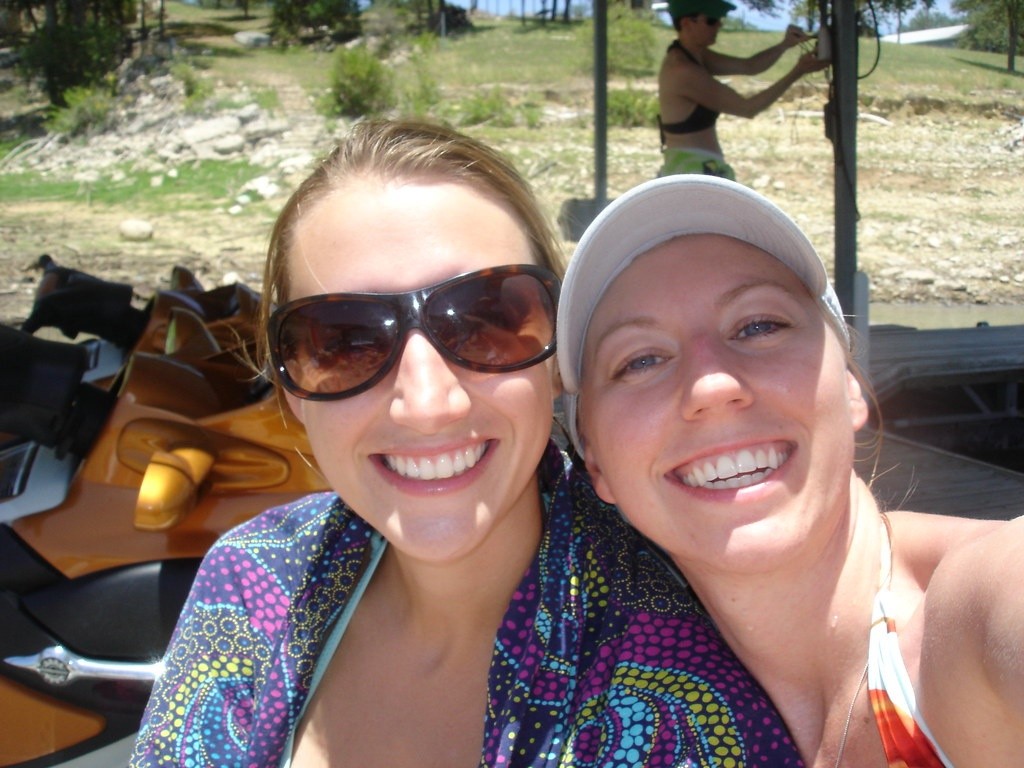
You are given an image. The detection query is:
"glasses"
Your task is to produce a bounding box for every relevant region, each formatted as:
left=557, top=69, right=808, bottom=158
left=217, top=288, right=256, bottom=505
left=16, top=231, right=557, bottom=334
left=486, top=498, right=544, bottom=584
left=264, top=262, right=562, bottom=398
left=704, top=17, right=718, bottom=25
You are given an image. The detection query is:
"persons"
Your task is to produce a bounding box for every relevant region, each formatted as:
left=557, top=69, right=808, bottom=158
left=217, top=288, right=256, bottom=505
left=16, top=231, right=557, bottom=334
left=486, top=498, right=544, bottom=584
left=129, top=119, right=803, bottom=767
left=556, top=174, right=1024, bottom=768
left=658, top=0, right=829, bottom=180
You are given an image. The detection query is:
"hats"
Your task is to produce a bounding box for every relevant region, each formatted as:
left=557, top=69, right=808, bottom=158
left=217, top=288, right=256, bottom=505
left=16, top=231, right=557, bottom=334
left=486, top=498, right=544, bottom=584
left=553, top=174, right=852, bottom=461
left=662, top=0, right=738, bottom=18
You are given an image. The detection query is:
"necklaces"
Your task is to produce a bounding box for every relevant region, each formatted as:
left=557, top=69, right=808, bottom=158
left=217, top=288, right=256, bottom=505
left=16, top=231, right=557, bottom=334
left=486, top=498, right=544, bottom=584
left=834, top=660, right=869, bottom=768
